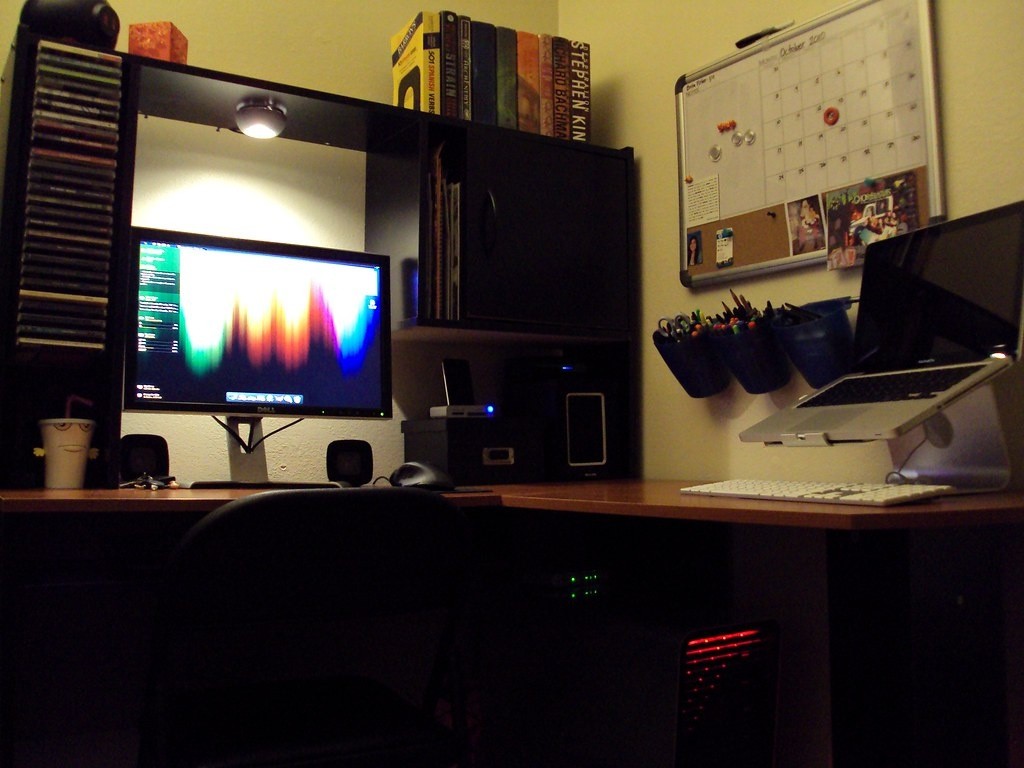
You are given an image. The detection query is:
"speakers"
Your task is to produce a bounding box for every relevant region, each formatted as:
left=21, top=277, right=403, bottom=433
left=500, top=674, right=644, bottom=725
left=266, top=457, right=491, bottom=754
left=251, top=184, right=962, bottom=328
left=326, top=439, right=374, bottom=488
left=120, top=434, right=169, bottom=484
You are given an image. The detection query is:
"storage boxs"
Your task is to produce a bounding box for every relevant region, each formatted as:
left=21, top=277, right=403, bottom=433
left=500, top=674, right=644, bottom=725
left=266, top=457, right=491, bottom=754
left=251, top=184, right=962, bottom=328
left=651, top=299, right=855, bottom=400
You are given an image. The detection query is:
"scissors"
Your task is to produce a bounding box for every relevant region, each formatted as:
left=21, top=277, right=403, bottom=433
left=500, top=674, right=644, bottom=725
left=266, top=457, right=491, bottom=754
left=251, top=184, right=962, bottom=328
left=658, top=314, right=690, bottom=337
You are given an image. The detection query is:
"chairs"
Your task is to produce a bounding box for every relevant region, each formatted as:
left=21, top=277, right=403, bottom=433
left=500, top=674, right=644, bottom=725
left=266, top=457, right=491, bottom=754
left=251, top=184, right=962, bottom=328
left=129, top=488, right=481, bottom=768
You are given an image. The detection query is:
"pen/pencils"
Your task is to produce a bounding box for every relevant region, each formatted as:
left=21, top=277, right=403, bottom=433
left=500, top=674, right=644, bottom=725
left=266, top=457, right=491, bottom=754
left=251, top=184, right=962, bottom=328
left=663, top=289, right=824, bottom=337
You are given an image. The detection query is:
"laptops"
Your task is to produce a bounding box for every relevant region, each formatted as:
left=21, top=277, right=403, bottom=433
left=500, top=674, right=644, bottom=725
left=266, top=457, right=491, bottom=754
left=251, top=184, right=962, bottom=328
left=738, top=197, right=1023, bottom=450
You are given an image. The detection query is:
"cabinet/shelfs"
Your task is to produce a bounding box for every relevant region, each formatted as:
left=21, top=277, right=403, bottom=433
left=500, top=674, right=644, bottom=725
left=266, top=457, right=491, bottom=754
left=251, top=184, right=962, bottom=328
left=8, top=22, right=642, bottom=483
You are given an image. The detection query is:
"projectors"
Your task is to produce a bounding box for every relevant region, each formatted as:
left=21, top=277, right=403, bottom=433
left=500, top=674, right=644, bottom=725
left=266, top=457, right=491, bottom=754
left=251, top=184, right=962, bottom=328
left=429, top=404, right=495, bottom=419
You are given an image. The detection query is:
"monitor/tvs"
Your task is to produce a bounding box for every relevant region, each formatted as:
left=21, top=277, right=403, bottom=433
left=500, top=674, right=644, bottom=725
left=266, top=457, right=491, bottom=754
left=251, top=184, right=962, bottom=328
left=121, top=225, right=394, bottom=422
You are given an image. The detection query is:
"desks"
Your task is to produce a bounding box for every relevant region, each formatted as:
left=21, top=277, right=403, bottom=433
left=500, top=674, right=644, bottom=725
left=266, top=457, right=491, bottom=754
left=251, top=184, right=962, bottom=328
left=1, top=480, right=1024, bottom=768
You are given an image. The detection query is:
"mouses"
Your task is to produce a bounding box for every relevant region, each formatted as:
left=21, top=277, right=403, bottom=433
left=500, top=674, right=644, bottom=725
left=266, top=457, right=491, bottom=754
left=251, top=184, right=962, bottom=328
left=388, top=460, right=455, bottom=492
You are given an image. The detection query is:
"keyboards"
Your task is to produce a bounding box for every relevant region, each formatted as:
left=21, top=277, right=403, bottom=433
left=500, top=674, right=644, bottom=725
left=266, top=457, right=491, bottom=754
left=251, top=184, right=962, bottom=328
left=680, top=478, right=958, bottom=508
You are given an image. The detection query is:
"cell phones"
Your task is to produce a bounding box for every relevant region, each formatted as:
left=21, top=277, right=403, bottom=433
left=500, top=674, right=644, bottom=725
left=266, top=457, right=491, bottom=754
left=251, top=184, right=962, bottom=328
left=442, top=358, right=476, bottom=406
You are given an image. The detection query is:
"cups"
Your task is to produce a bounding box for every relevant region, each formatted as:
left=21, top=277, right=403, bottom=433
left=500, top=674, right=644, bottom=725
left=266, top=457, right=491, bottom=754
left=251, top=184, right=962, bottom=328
left=33, top=418, right=100, bottom=492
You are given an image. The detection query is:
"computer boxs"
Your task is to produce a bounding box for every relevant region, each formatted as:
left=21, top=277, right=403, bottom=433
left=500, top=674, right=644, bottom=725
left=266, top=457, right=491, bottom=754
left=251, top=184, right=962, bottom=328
left=548, top=621, right=781, bottom=768
